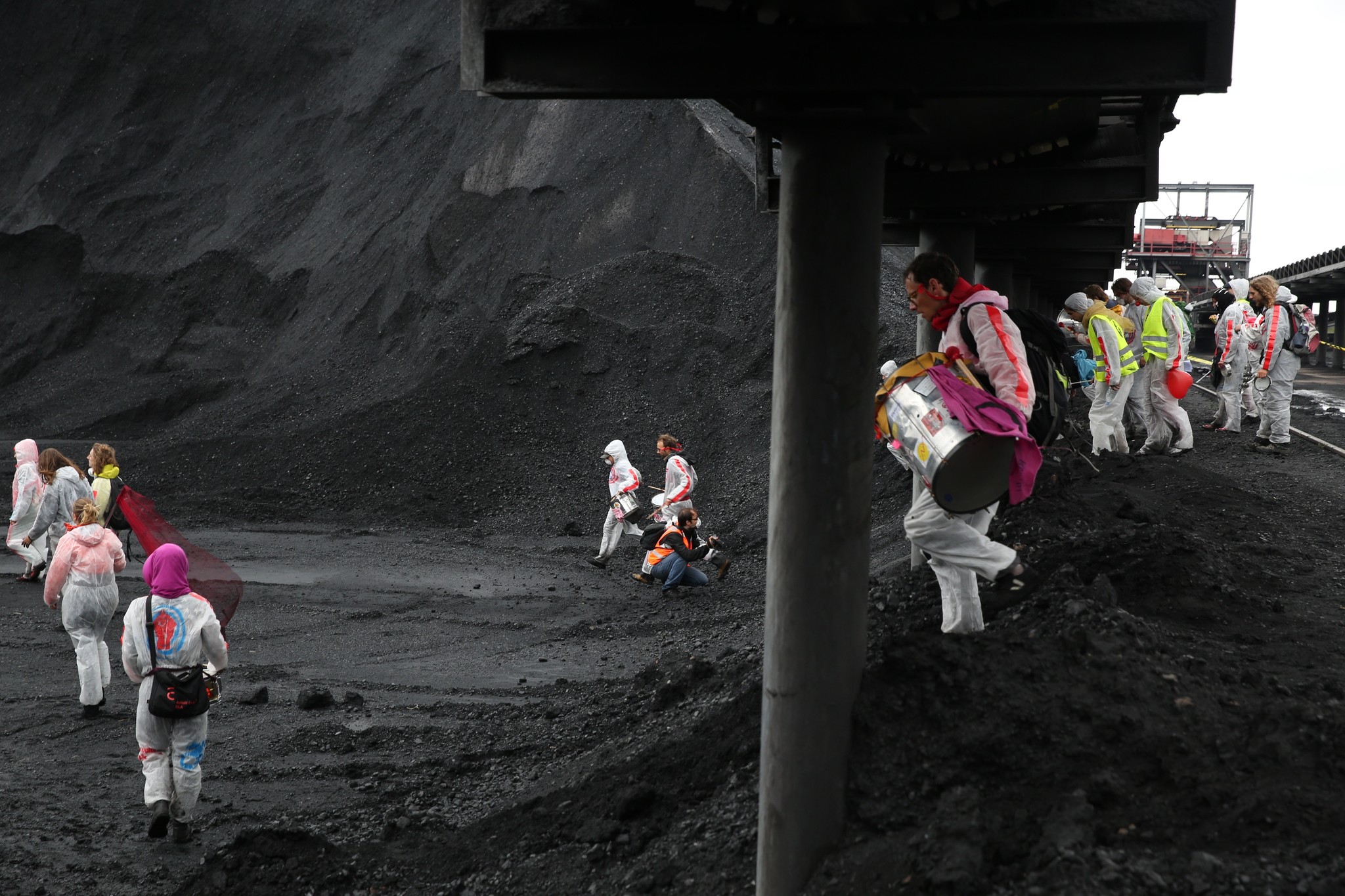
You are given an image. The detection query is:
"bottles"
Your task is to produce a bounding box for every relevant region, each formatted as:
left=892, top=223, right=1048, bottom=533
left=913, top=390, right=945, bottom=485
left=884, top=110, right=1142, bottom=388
left=1105, top=378, right=1123, bottom=406
left=1294, top=322, right=1310, bottom=348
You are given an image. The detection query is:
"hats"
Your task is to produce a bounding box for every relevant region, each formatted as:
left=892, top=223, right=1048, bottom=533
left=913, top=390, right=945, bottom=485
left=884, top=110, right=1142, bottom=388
left=600, top=452, right=610, bottom=459
left=1212, top=288, right=1234, bottom=308
left=1222, top=282, right=1232, bottom=289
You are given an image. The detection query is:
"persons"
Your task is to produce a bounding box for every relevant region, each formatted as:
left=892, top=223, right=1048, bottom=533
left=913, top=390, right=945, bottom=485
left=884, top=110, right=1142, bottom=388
left=642, top=508, right=708, bottom=602
left=21, top=447, right=95, bottom=559
left=120, top=543, right=228, bottom=843
left=87, top=443, right=126, bottom=533
left=44, top=496, right=126, bottom=715
left=1063, top=275, right=1301, bottom=459
left=6, top=440, right=49, bottom=583
left=630, top=435, right=732, bottom=586
left=585, top=441, right=645, bottom=570
left=894, top=250, right=1036, bottom=635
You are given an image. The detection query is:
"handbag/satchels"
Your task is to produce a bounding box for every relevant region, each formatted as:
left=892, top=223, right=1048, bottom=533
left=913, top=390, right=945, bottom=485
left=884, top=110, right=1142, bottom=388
left=1071, top=349, right=1096, bottom=387
left=1210, top=347, right=1224, bottom=390
left=148, top=663, right=210, bottom=719
left=624, top=493, right=644, bottom=524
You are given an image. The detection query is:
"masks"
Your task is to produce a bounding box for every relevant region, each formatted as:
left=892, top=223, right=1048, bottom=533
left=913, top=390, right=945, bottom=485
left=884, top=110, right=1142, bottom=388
left=1116, top=296, right=1125, bottom=307
left=604, top=458, right=613, bottom=465
left=695, top=518, right=701, bottom=529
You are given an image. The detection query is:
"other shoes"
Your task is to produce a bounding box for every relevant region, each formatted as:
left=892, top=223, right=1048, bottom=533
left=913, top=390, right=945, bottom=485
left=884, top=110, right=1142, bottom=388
left=16, top=575, right=38, bottom=583
left=987, top=563, right=1043, bottom=617
left=98, top=686, right=107, bottom=706
left=80, top=704, right=100, bottom=720
left=147, top=800, right=170, bottom=838
left=585, top=557, right=731, bottom=600
left=1134, top=415, right=1289, bottom=457
left=172, top=821, right=192, bottom=844
left=30, top=563, right=47, bottom=578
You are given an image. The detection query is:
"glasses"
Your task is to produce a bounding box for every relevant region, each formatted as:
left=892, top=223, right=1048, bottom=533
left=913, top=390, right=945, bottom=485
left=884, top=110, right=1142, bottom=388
left=691, top=517, right=698, bottom=520
left=656, top=448, right=661, bottom=451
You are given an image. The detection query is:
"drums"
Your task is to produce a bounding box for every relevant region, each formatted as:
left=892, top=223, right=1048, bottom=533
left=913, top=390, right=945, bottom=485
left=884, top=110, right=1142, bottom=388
left=1058, top=303, right=1093, bottom=346
left=199, top=662, right=222, bottom=706
left=876, top=351, right=1020, bottom=515
left=1254, top=374, right=1272, bottom=392
left=609, top=491, right=642, bottom=519
left=1220, top=362, right=1232, bottom=377
left=652, top=492, right=668, bottom=524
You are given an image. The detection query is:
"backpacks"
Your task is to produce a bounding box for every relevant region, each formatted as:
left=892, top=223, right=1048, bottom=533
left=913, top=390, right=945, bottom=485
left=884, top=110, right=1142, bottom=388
left=1174, top=301, right=1195, bottom=340
left=1261, top=300, right=1320, bottom=358
left=640, top=521, right=683, bottom=551
left=103, top=476, right=133, bottom=530
left=959, top=301, right=1072, bottom=455
